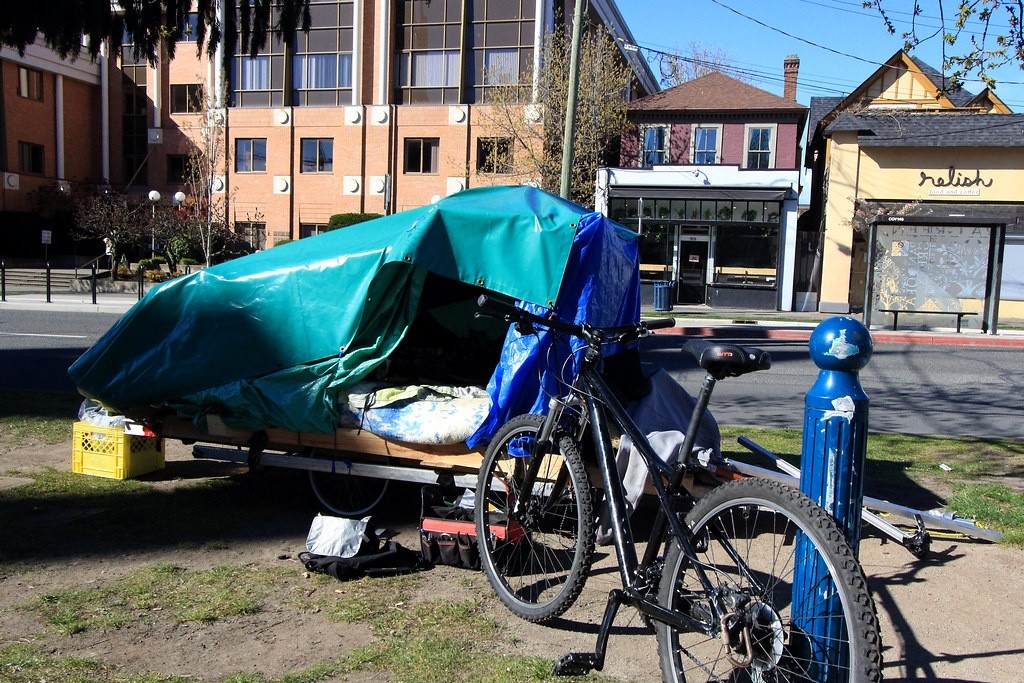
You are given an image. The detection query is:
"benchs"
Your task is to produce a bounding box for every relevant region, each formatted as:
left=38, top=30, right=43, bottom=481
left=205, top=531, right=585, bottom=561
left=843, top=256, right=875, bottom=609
left=879, top=310, right=978, bottom=333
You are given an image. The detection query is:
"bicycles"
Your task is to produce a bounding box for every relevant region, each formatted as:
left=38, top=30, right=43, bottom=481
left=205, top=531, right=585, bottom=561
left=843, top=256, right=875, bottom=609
left=471, top=293, right=885, bottom=683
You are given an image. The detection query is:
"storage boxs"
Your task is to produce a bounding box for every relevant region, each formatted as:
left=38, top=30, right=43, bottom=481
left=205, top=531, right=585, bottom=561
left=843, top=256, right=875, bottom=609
left=72, top=421, right=165, bottom=479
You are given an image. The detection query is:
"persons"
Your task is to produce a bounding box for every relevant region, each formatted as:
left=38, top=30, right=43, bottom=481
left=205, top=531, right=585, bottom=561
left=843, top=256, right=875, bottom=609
left=103, top=238, right=114, bottom=270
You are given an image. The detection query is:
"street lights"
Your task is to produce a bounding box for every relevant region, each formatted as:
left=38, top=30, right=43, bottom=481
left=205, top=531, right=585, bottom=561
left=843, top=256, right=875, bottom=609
left=175, top=192, right=186, bottom=213
left=148, top=191, right=160, bottom=258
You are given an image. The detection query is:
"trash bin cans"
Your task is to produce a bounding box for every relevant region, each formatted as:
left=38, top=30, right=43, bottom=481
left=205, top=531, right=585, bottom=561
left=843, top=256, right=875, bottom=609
left=654, top=281, right=673, bottom=311
